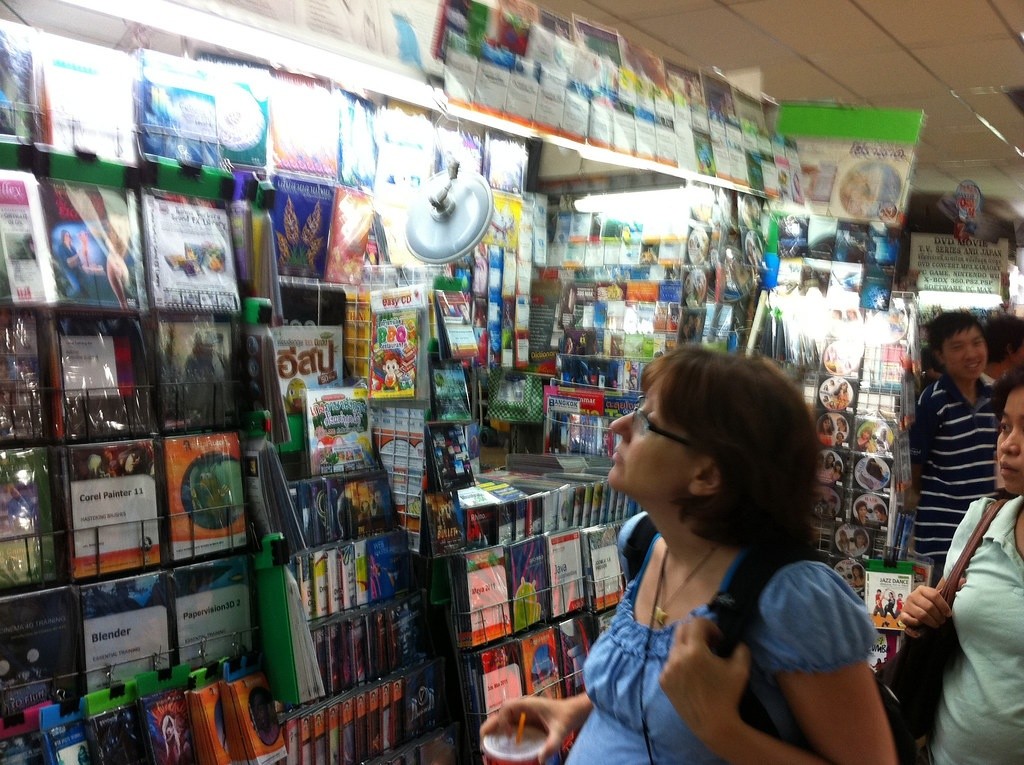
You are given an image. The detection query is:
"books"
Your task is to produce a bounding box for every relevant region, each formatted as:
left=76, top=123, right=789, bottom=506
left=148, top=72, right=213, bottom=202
left=445, top=472, right=645, bottom=714
left=864, top=571, right=914, bottom=631
left=0, top=30, right=252, bottom=729
left=286, top=468, right=442, bottom=765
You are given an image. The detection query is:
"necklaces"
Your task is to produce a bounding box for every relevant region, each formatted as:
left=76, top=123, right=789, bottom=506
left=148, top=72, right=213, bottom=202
left=649, top=541, right=718, bottom=626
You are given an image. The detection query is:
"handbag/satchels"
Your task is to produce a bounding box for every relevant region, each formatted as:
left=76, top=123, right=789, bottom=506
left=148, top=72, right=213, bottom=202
left=875, top=615, right=964, bottom=765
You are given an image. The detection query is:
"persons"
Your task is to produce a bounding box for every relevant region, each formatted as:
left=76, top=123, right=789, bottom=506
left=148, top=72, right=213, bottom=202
left=479, top=345, right=898, bottom=765
left=909, top=310, right=1024, bottom=563
left=899, top=368, right=1024, bottom=765
left=395, top=610, right=427, bottom=668
left=872, top=589, right=905, bottom=619
left=817, top=308, right=890, bottom=588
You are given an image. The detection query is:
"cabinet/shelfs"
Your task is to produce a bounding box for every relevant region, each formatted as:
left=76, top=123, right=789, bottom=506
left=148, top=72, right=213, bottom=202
left=0, top=21, right=916, bottom=765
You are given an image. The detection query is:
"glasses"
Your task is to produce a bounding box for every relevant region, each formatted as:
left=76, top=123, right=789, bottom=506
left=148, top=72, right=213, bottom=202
left=633, top=394, right=688, bottom=447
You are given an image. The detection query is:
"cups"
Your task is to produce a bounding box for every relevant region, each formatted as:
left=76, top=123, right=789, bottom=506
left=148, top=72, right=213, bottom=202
left=483, top=725, right=547, bottom=765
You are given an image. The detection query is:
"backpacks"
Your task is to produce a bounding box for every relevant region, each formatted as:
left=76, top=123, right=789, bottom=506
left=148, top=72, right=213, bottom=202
left=622, top=512, right=917, bottom=764
